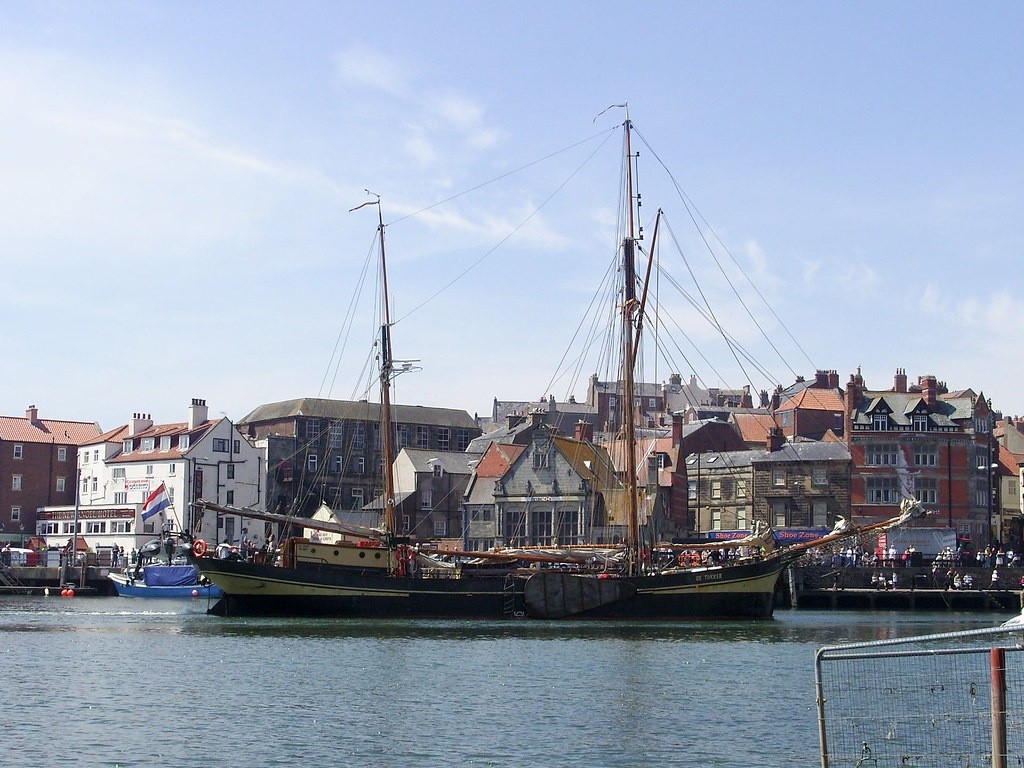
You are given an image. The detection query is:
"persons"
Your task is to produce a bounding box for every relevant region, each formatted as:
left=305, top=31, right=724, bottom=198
left=931, top=544, right=1024, bottom=592
left=805, top=545, right=916, bottom=591
left=653, top=546, right=765, bottom=565
left=215, top=528, right=277, bottom=565
left=182, top=529, right=191, bottom=544
left=1, top=544, right=11, bottom=553
left=110, top=543, right=144, bottom=567
left=590, top=554, right=600, bottom=565
left=414, top=543, right=461, bottom=563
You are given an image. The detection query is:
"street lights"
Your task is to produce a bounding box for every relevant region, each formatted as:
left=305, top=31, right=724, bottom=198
left=978, top=463, right=998, bottom=549
left=20, top=523, right=25, bottom=548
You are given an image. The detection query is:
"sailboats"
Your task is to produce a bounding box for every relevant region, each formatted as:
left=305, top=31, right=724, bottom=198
left=178, top=100, right=930, bottom=622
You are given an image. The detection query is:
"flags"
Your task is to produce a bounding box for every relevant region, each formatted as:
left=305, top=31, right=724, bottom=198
left=140, top=483, right=171, bottom=522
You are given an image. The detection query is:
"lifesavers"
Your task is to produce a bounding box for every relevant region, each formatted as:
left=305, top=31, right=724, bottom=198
left=192, top=540, right=207, bottom=558
left=396, top=546, right=414, bottom=563
left=639, top=544, right=650, bottom=563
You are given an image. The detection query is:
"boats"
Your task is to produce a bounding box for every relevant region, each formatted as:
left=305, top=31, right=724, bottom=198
left=108, top=521, right=225, bottom=598
